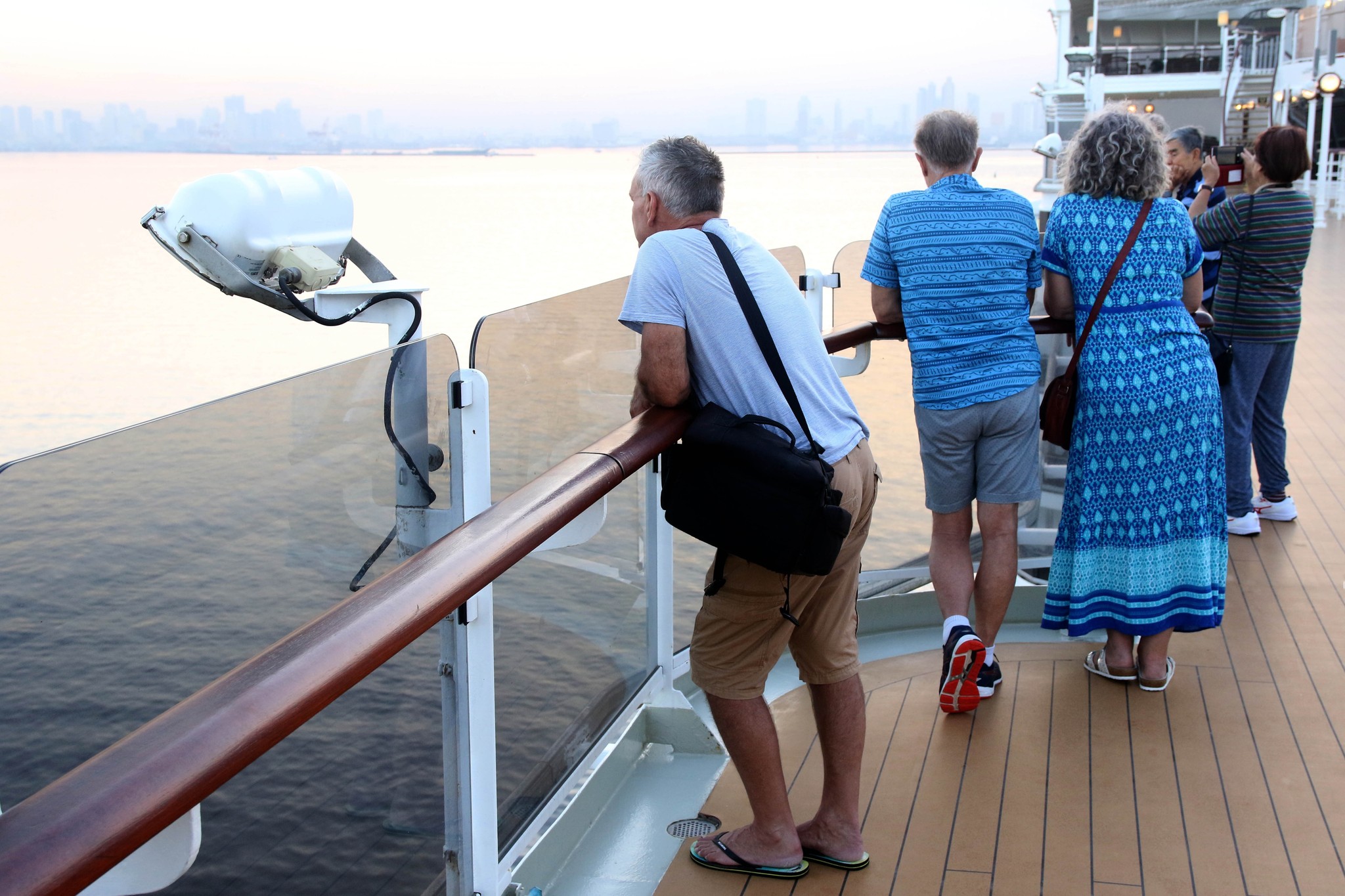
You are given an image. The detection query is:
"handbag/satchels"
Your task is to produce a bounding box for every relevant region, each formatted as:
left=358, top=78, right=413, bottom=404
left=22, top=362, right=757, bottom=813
left=661, top=401, right=852, bottom=577
left=1040, top=374, right=1077, bottom=451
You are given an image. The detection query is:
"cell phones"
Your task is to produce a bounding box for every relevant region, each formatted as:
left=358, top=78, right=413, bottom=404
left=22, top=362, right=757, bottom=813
left=1211, top=144, right=1244, bottom=166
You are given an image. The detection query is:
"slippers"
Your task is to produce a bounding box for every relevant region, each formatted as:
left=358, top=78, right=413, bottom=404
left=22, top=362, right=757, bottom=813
left=690, top=832, right=810, bottom=879
left=1136, top=656, right=1175, bottom=691
left=1083, top=645, right=1138, bottom=680
left=803, top=850, right=870, bottom=871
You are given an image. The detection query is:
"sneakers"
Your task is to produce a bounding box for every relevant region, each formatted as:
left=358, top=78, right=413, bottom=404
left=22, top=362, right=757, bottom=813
left=1251, top=495, right=1297, bottom=521
left=976, top=660, right=1002, bottom=699
left=1227, top=511, right=1261, bottom=536
left=939, top=625, right=986, bottom=713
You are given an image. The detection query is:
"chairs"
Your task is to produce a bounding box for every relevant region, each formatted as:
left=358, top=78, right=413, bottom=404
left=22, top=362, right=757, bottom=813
left=1105, top=52, right=1204, bottom=74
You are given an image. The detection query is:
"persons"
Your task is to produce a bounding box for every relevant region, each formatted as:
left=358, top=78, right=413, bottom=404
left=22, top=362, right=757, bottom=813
left=1162, top=125, right=1232, bottom=313
left=617, top=133, right=884, bottom=881
left=1187, top=124, right=1315, bottom=536
left=858, top=108, right=1045, bottom=719
left=1040, top=101, right=1229, bottom=693
left=1202, top=133, right=1221, bottom=163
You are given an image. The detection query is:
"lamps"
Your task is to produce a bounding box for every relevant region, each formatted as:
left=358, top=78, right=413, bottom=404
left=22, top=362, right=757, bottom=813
left=1266, top=7, right=1289, bottom=18
left=1217, top=9, right=1229, bottom=28
left=1086, top=16, right=1093, bottom=31
left=1113, top=25, right=1123, bottom=38
left=136, top=166, right=443, bottom=557
left=1031, top=131, right=1064, bottom=159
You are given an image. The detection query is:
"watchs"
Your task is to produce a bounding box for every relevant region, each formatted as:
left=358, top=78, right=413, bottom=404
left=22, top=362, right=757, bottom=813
left=1197, top=183, right=1214, bottom=195
